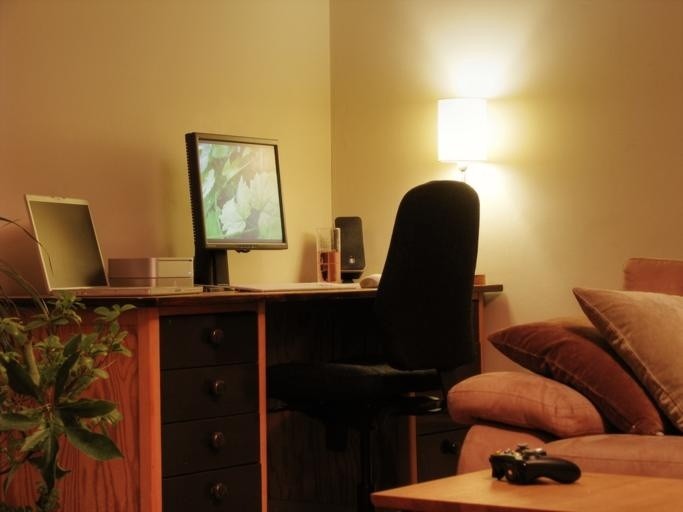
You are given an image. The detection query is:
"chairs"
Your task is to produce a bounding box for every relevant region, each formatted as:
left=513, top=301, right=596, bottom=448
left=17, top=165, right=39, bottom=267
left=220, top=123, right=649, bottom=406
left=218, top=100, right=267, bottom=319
left=267, top=179, right=480, bottom=512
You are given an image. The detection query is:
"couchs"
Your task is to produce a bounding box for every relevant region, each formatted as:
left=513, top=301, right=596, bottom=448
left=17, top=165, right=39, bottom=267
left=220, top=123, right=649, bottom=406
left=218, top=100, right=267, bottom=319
left=448, top=258, right=683, bottom=477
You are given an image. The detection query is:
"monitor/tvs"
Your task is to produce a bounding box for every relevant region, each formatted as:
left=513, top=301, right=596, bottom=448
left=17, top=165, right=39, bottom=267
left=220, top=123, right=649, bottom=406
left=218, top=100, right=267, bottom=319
left=184, top=130, right=288, bottom=290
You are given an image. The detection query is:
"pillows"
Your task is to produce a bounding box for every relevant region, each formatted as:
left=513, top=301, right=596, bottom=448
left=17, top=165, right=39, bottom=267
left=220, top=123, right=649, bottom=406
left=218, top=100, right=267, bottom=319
left=572, top=287, right=683, bottom=436
left=446, top=371, right=604, bottom=438
left=487, top=316, right=666, bottom=436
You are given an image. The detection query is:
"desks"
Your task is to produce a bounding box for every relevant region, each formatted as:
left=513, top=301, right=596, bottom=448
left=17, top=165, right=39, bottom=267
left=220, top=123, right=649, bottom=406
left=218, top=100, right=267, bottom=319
left=0, top=283, right=502, bottom=512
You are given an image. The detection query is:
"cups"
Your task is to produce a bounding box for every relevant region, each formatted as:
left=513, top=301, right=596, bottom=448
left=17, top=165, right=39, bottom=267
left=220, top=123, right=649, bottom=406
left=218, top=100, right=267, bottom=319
left=315, top=228, right=342, bottom=282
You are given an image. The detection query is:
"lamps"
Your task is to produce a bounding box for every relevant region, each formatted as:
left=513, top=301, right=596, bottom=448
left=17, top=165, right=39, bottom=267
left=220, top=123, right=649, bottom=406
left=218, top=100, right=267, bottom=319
left=438, top=98, right=486, bottom=181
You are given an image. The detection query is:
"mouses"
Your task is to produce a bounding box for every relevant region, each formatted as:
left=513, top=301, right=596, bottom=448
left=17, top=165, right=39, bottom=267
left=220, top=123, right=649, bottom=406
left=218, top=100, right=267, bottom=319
left=361, top=274, right=382, bottom=291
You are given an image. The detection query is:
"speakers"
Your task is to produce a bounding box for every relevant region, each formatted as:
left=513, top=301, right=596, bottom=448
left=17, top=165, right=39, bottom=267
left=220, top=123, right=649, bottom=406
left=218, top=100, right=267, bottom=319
left=335, top=215, right=366, bottom=283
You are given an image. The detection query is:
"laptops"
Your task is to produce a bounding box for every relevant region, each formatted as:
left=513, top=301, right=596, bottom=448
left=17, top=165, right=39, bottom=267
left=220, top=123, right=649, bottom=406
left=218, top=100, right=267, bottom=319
left=24, top=193, right=204, bottom=295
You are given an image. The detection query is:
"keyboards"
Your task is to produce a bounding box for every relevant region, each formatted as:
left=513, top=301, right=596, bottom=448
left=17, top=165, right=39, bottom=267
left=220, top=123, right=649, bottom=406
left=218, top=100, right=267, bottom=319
left=237, top=279, right=362, bottom=293
